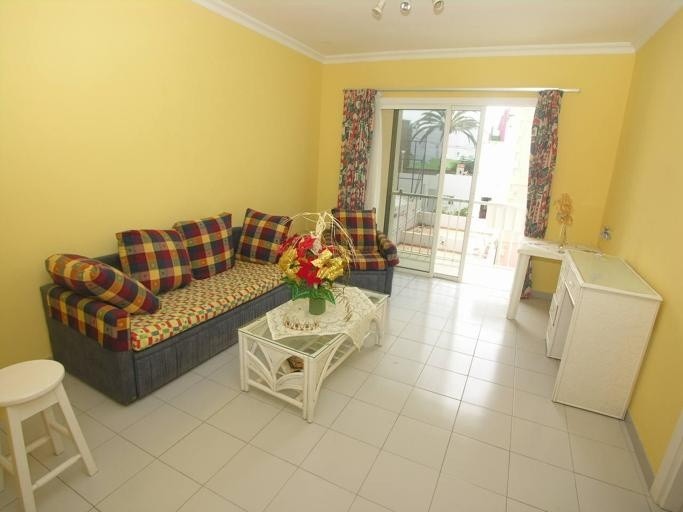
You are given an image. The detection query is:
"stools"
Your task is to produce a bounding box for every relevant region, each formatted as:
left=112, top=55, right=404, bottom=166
left=0, top=359, right=99, bottom=512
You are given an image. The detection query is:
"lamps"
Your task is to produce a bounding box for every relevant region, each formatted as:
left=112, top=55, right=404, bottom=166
left=370, top=0, right=446, bottom=20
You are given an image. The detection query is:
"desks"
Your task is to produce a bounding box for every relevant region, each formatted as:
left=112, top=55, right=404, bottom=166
left=505, top=237, right=608, bottom=320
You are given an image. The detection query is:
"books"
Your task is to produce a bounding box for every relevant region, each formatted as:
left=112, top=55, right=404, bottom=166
left=282, top=355, right=305, bottom=375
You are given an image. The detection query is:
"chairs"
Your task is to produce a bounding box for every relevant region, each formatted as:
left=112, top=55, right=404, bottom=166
left=320, top=225, right=399, bottom=298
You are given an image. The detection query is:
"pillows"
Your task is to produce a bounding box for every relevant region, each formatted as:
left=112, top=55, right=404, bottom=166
left=45, top=207, right=294, bottom=315
left=331, top=206, right=378, bottom=249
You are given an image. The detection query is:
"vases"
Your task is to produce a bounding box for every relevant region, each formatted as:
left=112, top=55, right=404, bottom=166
left=308, top=284, right=326, bottom=315
left=556, top=222, right=570, bottom=249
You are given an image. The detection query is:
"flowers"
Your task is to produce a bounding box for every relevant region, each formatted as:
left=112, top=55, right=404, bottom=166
left=274, top=211, right=358, bottom=305
left=552, top=192, right=574, bottom=225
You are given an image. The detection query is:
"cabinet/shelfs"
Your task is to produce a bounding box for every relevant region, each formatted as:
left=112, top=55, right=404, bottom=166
left=544, top=248, right=663, bottom=420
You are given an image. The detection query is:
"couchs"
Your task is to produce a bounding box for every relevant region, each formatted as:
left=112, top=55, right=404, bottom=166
left=39, top=225, right=297, bottom=408
